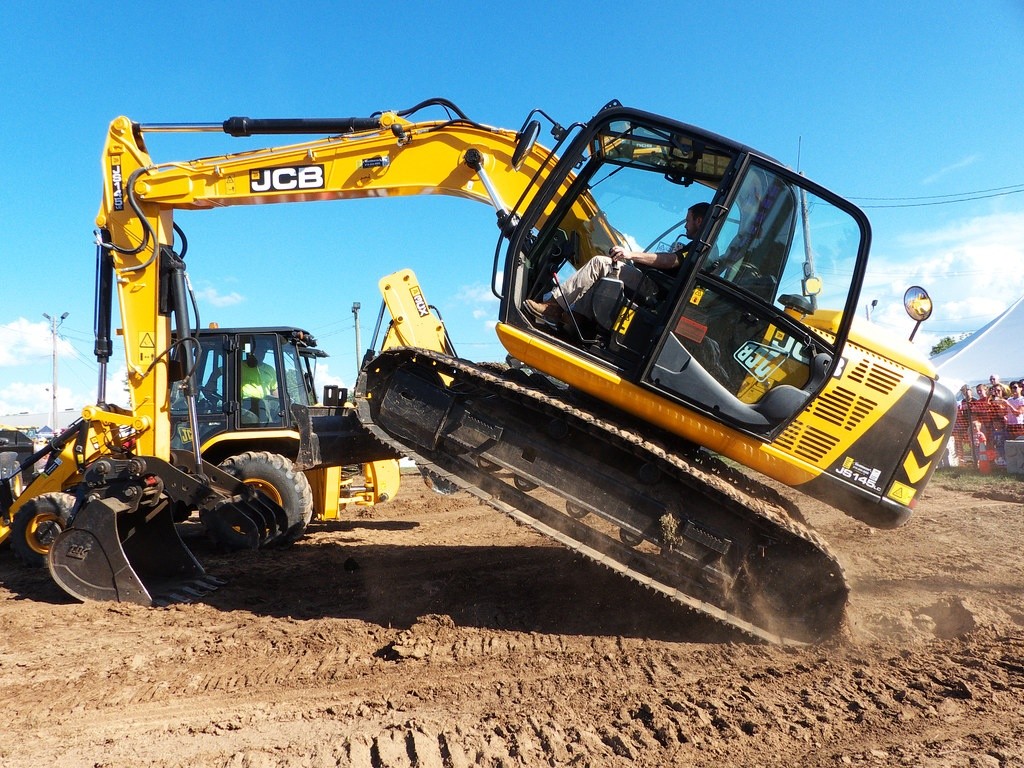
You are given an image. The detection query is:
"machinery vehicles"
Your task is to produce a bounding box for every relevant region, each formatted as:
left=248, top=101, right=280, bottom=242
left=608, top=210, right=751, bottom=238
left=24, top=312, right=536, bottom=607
left=0, top=95, right=956, bottom=650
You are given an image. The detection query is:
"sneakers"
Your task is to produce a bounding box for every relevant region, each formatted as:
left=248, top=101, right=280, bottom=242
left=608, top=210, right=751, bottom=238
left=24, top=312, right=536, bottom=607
left=562, top=311, right=574, bottom=325
left=524, top=300, right=562, bottom=320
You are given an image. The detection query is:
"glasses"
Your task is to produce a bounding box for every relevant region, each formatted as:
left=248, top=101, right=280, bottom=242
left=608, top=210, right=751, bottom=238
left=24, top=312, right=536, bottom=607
left=1020, top=384, right=1024, bottom=386
left=1011, top=386, right=1017, bottom=389
left=990, top=378, right=996, bottom=380
left=994, top=390, right=1001, bottom=392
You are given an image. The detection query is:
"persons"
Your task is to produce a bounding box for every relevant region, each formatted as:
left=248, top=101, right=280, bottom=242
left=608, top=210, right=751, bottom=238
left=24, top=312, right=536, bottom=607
left=205, top=339, right=283, bottom=424
left=523, top=202, right=714, bottom=330
left=950, top=373, right=1024, bottom=472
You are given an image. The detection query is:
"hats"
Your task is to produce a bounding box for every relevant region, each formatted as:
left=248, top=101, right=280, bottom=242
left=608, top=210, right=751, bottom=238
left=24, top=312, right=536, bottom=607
left=961, top=384, right=971, bottom=390
